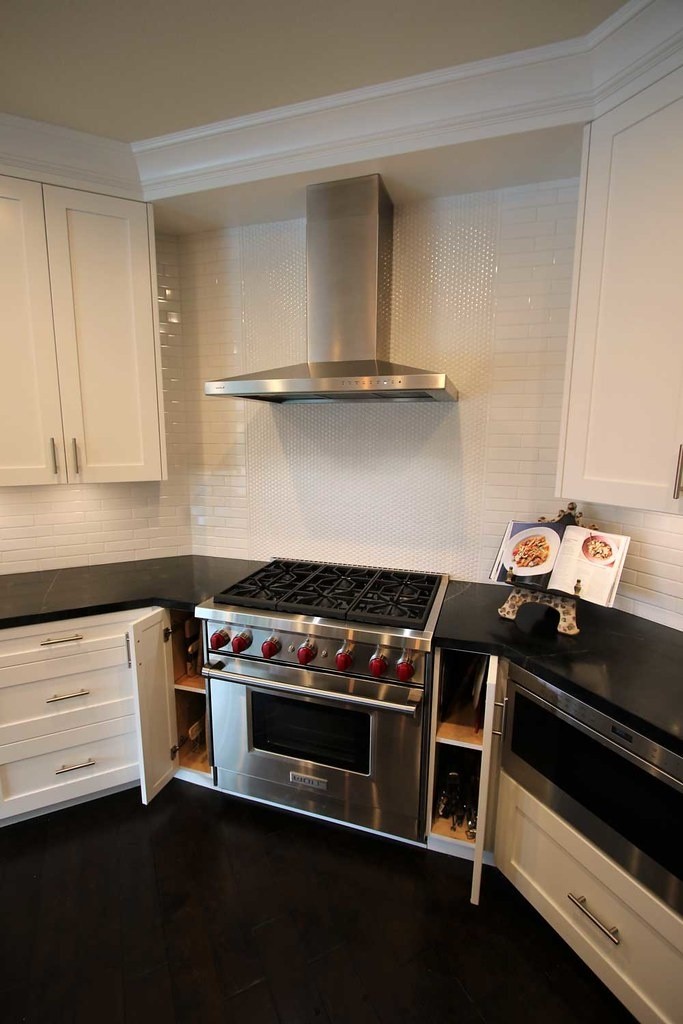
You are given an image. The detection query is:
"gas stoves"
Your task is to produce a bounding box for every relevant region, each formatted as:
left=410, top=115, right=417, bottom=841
left=194, top=556, right=450, bottom=689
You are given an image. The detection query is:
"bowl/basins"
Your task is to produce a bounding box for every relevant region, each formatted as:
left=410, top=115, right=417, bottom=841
left=582, top=535, right=618, bottom=565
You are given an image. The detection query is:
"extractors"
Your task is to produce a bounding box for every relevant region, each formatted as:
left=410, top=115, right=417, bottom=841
left=202, top=172, right=461, bottom=404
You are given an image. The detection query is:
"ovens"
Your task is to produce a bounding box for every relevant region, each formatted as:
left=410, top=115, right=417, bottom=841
left=198, top=668, right=425, bottom=842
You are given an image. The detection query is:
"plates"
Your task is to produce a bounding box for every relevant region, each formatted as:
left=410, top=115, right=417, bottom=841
left=503, top=527, right=560, bottom=576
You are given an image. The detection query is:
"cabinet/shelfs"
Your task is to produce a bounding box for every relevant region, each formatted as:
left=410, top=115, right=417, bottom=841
left=0, top=608, right=155, bottom=827
left=553, top=66, right=683, bottom=515
left=497, top=771, right=683, bottom=1023
left=0, top=174, right=168, bottom=486
left=430, top=654, right=507, bottom=906
left=133, top=607, right=209, bottom=805
